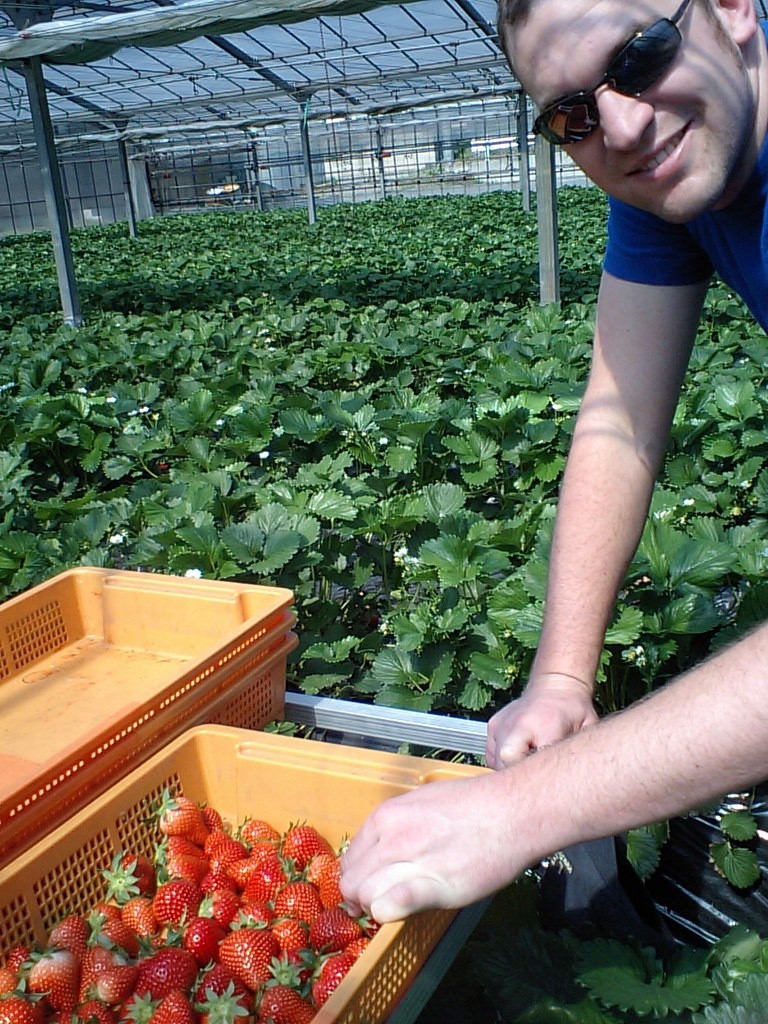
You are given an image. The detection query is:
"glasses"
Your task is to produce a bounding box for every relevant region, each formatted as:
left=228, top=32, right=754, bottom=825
left=531, top=0, right=690, bottom=147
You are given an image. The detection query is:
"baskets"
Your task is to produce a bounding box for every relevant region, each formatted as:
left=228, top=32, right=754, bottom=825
left=0, top=725, right=492, bottom=1024
left=0, top=546, right=300, bottom=865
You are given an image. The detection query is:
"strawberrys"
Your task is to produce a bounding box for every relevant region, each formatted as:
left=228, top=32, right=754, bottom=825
left=0, top=790, right=382, bottom=1024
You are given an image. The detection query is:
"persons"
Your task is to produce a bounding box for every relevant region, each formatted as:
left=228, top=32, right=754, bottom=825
left=339, top=0, right=768, bottom=924
